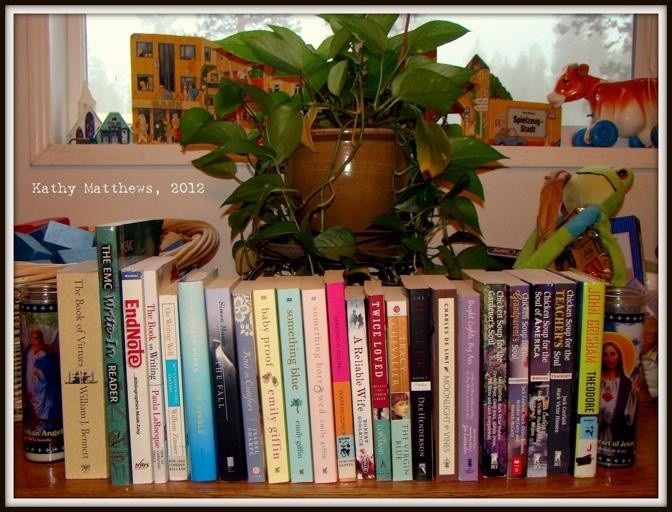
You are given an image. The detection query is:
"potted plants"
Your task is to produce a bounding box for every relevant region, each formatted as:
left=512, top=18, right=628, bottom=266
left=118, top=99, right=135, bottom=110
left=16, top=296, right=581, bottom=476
left=180, top=13, right=509, bottom=280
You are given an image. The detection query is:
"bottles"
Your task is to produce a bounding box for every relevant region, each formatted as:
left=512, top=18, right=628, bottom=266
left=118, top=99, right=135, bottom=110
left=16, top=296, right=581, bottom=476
left=20, top=286, right=65, bottom=461
left=596, top=286, right=646, bottom=467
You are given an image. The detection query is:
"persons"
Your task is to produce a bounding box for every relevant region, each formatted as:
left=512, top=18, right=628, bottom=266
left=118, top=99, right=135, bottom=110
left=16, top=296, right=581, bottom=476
left=25, top=326, right=60, bottom=432
left=598, top=329, right=643, bottom=458
left=64, top=79, right=103, bottom=144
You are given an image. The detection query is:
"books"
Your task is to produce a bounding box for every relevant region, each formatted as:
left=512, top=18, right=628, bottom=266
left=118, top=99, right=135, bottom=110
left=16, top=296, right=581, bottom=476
left=607, top=214, right=644, bottom=286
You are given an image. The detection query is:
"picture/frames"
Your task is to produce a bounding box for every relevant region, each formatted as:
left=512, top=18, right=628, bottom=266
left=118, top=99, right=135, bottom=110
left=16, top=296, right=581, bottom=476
left=490, top=99, right=561, bottom=146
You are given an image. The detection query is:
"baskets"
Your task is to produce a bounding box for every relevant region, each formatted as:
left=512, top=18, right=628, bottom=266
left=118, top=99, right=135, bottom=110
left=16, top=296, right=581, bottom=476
left=13, top=220, right=216, bottom=422
